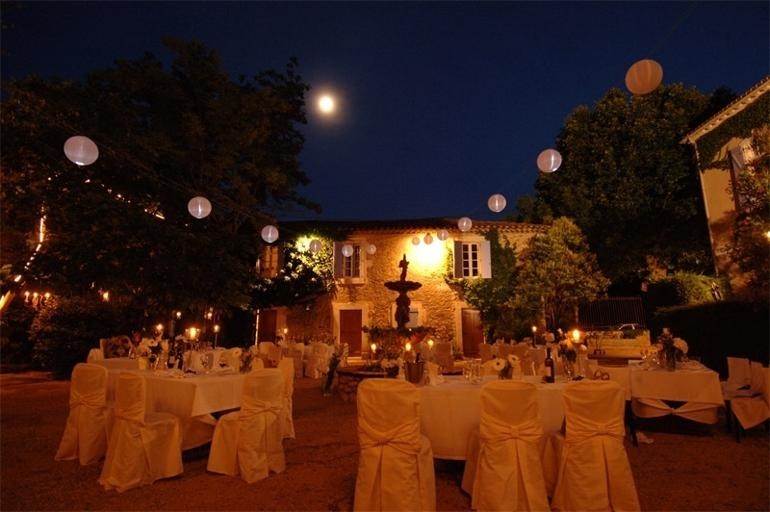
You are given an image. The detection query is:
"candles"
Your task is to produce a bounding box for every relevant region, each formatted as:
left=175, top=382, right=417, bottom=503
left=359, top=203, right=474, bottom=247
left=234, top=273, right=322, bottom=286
left=428, top=340, right=432, bottom=352
left=284, top=329, right=287, bottom=333
left=371, top=344, right=376, bottom=351
left=405, top=343, right=413, bottom=351
left=214, top=325, right=219, bottom=333
left=189, top=327, right=197, bottom=339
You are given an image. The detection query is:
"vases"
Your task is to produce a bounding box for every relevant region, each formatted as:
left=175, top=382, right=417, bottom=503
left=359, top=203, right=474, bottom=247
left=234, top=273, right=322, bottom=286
left=498, top=367, right=514, bottom=380
left=666, top=356, right=676, bottom=370
left=563, top=357, right=575, bottom=380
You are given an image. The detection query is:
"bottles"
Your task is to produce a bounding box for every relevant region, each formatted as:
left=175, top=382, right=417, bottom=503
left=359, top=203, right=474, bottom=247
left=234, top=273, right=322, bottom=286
left=544, top=347, right=555, bottom=382
left=173, top=339, right=183, bottom=369
left=167, top=337, right=175, bottom=368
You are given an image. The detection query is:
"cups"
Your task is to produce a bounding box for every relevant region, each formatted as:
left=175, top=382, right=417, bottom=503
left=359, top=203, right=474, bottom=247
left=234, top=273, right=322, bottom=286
left=472, top=359, right=482, bottom=385
left=640, top=348, right=661, bottom=371
left=576, top=353, right=590, bottom=378
left=463, top=362, right=474, bottom=386
left=585, top=360, right=601, bottom=379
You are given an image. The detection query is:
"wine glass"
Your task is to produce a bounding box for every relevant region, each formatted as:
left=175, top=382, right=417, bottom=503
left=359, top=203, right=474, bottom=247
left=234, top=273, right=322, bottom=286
left=146, top=354, right=159, bottom=374
left=564, top=358, right=575, bottom=384
left=218, top=358, right=229, bottom=377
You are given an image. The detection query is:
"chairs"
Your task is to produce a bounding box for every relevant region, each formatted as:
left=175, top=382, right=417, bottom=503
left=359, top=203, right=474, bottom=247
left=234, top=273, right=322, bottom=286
left=553, top=381, right=642, bottom=512
left=731, top=368, right=770, bottom=444
left=226, top=347, right=242, bottom=371
left=530, top=350, right=547, bottom=366
left=282, top=343, right=305, bottom=377
left=86, top=349, right=104, bottom=365
left=97, top=370, right=184, bottom=493
left=719, top=358, right=750, bottom=401
left=480, top=359, right=522, bottom=378
left=585, top=363, right=639, bottom=447
left=206, top=368, right=285, bottom=483
left=55, top=363, right=115, bottom=466
left=259, top=341, right=274, bottom=354
left=462, top=382, right=551, bottom=512
left=305, top=343, right=328, bottom=378
left=354, top=379, right=436, bottom=512
left=208, top=352, right=216, bottom=370
left=435, top=341, right=466, bottom=373
left=249, top=357, right=264, bottom=368
left=530, top=362, right=551, bottom=376
left=724, top=361, right=763, bottom=433
left=478, top=344, right=492, bottom=362
left=511, top=342, right=532, bottom=375
left=278, top=358, right=296, bottom=439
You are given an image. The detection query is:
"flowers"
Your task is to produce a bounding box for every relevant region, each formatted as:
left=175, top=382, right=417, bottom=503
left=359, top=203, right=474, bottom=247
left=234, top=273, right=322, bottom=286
left=492, top=354, right=519, bottom=372
left=657, top=327, right=685, bottom=364
left=558, top=340, right=578, bottom=365
